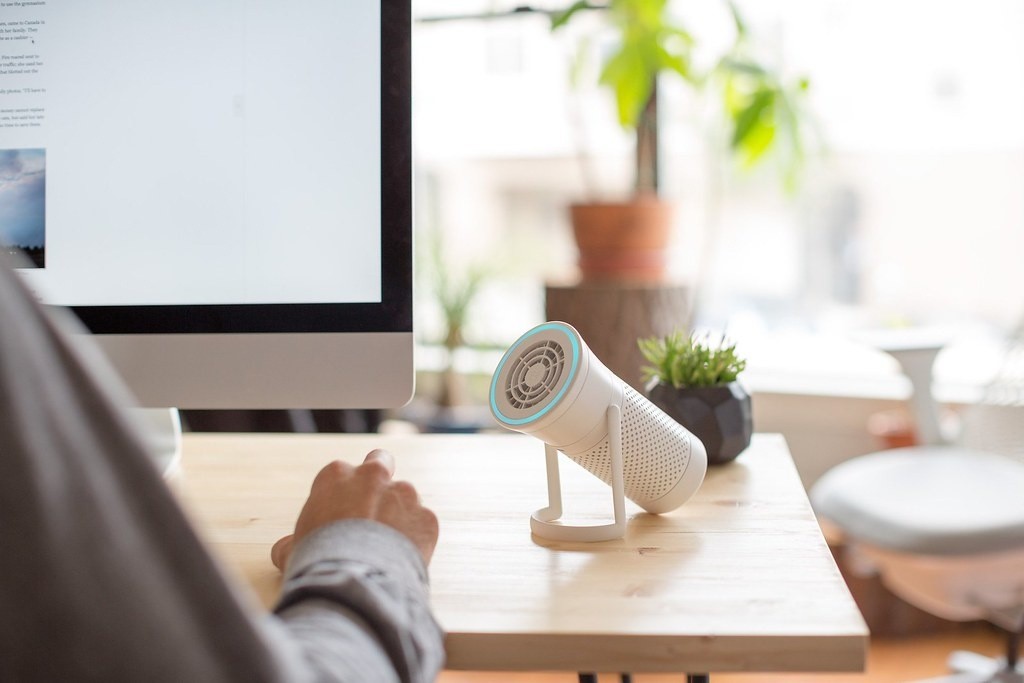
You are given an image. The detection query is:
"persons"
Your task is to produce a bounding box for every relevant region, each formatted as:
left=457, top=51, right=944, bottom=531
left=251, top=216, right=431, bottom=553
left=0, top=243, right=445, bottom=683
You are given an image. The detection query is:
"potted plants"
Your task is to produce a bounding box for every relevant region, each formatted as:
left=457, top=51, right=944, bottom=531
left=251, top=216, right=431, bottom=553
left=542, top=2, right=810, bottom=284
left=639, top=333, right=752, bottom=466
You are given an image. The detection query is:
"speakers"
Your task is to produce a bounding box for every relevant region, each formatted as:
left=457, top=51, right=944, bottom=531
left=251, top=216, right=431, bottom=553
left=487, top=318, right=707, bottom=542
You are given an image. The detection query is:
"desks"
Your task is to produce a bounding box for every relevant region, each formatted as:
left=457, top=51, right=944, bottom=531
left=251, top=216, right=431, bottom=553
left=167, top=431, right=871, bottom=683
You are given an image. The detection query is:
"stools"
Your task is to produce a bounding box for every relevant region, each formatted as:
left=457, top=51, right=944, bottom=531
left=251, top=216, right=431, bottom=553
left=813, top=445, right=1024, bottom=683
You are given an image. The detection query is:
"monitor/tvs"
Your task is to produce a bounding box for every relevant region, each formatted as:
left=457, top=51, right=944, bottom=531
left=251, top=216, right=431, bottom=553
left=0, top=0, right=412, bottom=485
